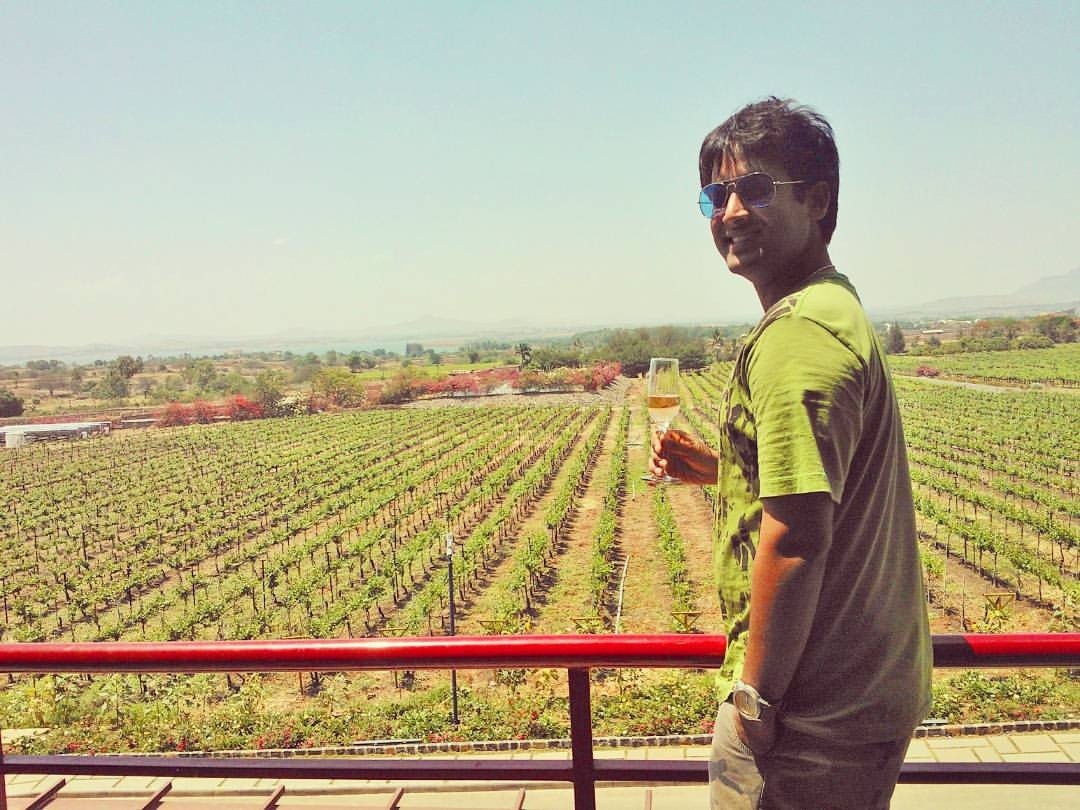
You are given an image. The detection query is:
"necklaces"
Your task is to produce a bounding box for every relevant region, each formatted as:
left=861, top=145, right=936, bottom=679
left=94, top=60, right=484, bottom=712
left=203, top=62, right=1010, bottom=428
left=787, top=265, right=835, bottom=293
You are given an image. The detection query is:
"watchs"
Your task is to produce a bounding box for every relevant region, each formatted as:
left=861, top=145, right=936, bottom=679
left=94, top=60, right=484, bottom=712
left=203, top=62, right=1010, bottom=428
left=725, top=684, right=784, bottom=721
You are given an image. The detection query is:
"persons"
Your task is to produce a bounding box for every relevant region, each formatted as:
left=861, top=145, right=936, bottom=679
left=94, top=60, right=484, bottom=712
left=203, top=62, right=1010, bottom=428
left=647, top=96, right=933, bottom=810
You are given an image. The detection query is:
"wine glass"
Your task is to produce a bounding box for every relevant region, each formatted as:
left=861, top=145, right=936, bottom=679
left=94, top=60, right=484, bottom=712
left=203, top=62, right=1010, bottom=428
left=640, top=359, right=683, bottom=489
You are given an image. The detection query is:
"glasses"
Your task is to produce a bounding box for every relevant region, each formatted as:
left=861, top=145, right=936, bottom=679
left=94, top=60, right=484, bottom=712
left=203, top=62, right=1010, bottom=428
left=697, top=172, right=811, bottom=218
